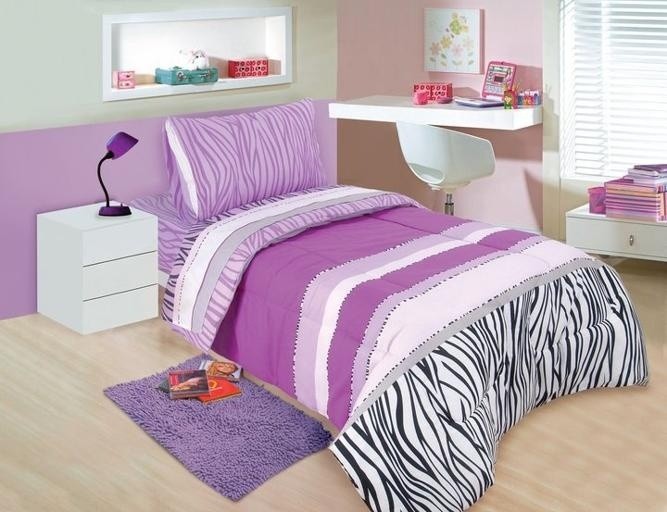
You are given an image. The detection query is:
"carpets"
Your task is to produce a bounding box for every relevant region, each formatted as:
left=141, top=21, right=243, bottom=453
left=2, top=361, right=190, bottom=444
left=102, top=353, right=334, bottom=503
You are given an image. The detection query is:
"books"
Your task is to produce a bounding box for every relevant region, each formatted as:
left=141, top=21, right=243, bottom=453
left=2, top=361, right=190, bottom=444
left=622, top=163, right=667, bottom=186
left=154, top=358, right=247, bottom=406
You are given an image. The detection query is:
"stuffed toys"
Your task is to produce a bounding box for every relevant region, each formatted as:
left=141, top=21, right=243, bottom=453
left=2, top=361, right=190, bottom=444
left=168, top=45, right=210, bottom=72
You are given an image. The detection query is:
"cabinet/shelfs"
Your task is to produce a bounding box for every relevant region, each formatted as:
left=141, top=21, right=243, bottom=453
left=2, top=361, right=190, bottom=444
left=36, top=200, right=159, bottom=336
left=566, top=203, right=667, bottom=262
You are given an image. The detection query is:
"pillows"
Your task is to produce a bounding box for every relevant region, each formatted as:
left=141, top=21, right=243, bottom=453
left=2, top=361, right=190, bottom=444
left=162, top=98, right=329, bottom=225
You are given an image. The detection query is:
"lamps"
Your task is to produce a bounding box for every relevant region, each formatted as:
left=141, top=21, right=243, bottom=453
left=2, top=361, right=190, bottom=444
left=97, top=131, right=139, bottom=216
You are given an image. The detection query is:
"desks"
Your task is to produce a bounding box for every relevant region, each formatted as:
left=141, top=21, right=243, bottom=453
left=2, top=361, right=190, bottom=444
left=328, top=94, right=544, bottom=131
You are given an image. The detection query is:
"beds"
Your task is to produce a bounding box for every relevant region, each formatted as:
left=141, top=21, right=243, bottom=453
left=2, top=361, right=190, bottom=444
left=129, top=183, right=649, bottom=512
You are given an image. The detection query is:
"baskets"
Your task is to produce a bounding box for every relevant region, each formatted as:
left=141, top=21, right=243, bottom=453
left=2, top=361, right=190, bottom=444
left=153, top=66, right=220, bottom=86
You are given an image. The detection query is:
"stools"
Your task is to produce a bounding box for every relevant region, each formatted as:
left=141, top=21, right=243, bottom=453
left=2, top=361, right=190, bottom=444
left=395, top=121, right=495, bottom=216
